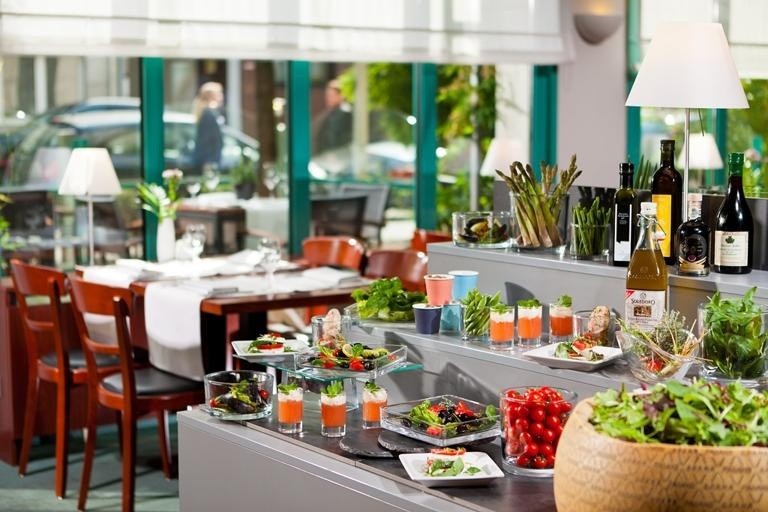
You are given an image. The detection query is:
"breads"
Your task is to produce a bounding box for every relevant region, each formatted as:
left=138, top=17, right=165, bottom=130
left=588, top=304, right=610, bottom=333
left=322, top=309, right=342, bottom=337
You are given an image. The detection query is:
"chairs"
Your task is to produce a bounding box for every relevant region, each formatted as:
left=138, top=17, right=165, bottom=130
left=411, top=225, right=451, bottom=251
left=297, top=231, right=365, bottom=329
left=9, top=177, right=395, bottom=255
left=341, top=247, right=431, bottom=312
left=9, top=257, right=140, bottom=503
left=64, top=271, right=203, bottom=511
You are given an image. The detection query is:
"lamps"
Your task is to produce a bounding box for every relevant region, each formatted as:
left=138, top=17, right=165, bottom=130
left=675, top=131, right=723, bottom=189
left=624, top=20, right=750, bottom=223
left=25, top=146, right=74, bottom=221
left=573, top=1, right=623, bottom=44
left=480, top=138, right=531, bottom=214
left=57, top=147, right=122, bottom=265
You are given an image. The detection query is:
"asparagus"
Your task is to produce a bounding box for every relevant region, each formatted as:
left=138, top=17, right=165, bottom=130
left=495, top=152, right=583, bottom=248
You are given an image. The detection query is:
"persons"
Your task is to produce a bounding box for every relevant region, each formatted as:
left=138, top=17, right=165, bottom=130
left=316, top=78, right=353, bottom=154
left=189, top=80, right=227, bottom=170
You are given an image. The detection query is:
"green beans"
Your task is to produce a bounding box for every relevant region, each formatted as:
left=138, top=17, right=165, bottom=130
left=458, top=287, right=502, bottom=336
left=428, top=454, right=463, bottom=476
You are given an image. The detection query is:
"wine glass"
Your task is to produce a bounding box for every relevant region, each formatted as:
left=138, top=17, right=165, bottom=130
left=257, top=239, right=281, bottom=292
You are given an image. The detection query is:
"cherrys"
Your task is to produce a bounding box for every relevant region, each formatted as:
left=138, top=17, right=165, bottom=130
left=501, top=387, right=571, bottom=468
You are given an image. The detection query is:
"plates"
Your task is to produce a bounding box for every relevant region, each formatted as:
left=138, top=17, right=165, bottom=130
left=398, top=450, right=505, bottom=488
left=232, top=335, right=309, bottom=365
left=523, top=340, right=622, bottom=372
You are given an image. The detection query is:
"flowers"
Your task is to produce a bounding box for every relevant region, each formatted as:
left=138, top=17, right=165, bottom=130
left=135, top=168, right=184, bottom=220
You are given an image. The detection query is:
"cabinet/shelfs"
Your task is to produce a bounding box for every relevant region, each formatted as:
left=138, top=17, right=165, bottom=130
left=175, top=179, right=767, bottom=512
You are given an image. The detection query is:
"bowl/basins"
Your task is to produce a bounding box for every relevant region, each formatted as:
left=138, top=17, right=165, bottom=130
left=204, top=370, right=274, bottom=420
left=452, top=210, right=511, bottom=249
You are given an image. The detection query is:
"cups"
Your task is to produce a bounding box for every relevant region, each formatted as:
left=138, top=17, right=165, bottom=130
left=322, top=395, right=347, bottom=438
left=570, top=223, right=610, bottom=262
left=412, top=269, right=490, bottom=342
left=509, top=190, right=570, bottom=252
left=491, top=303, right=616, bottom=349
left=277, top=388, right=303, bottom=434
left=362, top=390, right=387, bottom=428
left=185, top=223, right=207, bottom=263
left=311, top=316, right=351, bottom=344
left=501, top=385, right=578, bottom=478
left=697, top=301, right=767, bottom=389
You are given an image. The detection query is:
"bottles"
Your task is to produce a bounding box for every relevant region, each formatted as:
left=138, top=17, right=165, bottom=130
left=615, top=138, right=755, bottom=351
